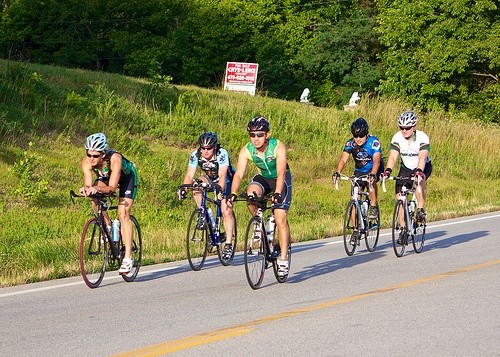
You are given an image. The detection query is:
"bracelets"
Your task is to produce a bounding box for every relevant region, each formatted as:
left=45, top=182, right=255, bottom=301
left=386, top=168, right=392, bottom=173
left=417, top=168, right=422, bottom=172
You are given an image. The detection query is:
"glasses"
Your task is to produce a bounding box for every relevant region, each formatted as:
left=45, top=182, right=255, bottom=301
left=201, top=146, right=214, bottom=150
left=86, top=154, right=101, bottom=159
left=250, top=132, right=266, bottom=138
left=353, top=135, right=366, bottom=138
left=400, top=127, right=413, bottom=131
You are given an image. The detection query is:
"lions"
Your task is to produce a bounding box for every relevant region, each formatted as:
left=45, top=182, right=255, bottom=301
left=299, top=87, right=310, bottom=103
left=348, top=91, right=361, bottom=106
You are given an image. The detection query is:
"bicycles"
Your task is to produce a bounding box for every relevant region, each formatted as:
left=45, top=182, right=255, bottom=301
left=178, top=178, right=237, bottom=271
left=335, top=172, right=380, bottom=256
left=381, top=171, right=427, bottom=258
left=233, top=191, right=291, bottom=290
left=69, top=190, right=142, bottom=289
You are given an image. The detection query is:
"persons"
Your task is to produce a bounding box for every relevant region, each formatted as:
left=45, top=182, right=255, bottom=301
left=332, top=118, right=384, bottom=245
left=80, top=133, right=138, bottom=275
left=178, top=132, right=236, bottom=260
left=227, top=117, right=292, bottom=278
left=384, top=111, right=432, bottom=244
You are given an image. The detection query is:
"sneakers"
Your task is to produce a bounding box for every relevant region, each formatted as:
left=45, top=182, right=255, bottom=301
left=222, top=245, right=233, bottom=260
left=253, top=227, right=261, bottom=243
left=277, top=254, right=289, bottom=277
left=369, top=207, right=378, bottom=218
left=350, top=230, right=358, bottom=246
left=101, top=219, right=112, bottom=243
left=119, top=257, right=134, bottom=273
left=196, top=217, right=205, bottom=229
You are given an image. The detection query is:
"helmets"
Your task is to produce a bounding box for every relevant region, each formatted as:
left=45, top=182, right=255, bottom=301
left=198, top=132, right=217, bottom=147
left=398, top=111, right=417, bottom=127
left=85, top=133, right=107, bottom=151
left=351, top=118, right=368, bottom=134
left=247, top=117, right=269, bottom=133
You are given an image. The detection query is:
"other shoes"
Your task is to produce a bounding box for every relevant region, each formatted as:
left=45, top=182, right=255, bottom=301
left=416, top=210, right=425, bottom=221
left=397, top=234, right=407, bottom=245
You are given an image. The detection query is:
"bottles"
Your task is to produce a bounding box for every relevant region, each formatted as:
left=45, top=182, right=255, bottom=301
left=407, top=202, right=413, bottom=217
left=266, top=215, right=276, bottom=242
left=358, top=200, right=366, bottom=217
left=111, top=218, right=119, bottom=241
left=409, top=199, right=416, bottom=217
left=363, top=200, right=369, bottom=217
left=207, top=208, right=216, bottom=229
